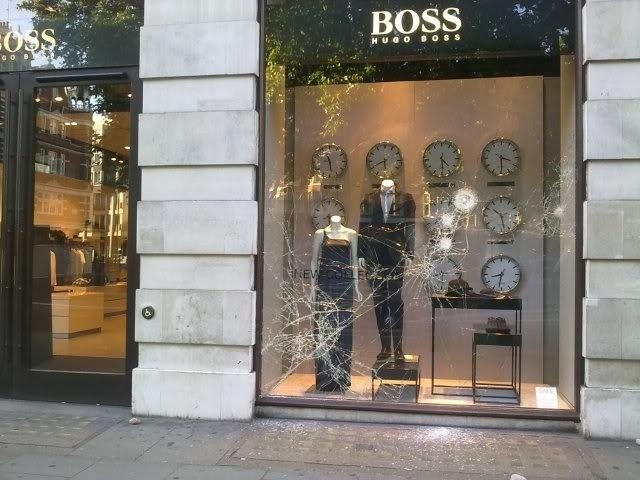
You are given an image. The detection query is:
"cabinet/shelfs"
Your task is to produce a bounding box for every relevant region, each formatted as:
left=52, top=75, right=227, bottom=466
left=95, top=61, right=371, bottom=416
left=54, top=282, right=127, bottom=317
left=429, top=293, right=523, bottom=398
left=471, top=332, right=524, bottom=407
left=368, top=350, right=422, bottom=403
left=52, top=291, right=105, bottom=340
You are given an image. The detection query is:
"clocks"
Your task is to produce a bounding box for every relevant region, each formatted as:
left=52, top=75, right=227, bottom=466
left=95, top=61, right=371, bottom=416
left=478, top=137, right=523, bottom=177
left=422, top=195, right=463, bottom=239
left=482, top=196, right=524, bottom=235
left=311, top=141, right=349, bottom=181
left=366, top=140, right=404, bottom=180
left=422, top=139, right=464, bottom=177
left=480, top=255, right=522, bottom=295
left=310, top=197, right=348, bottom=232
left=427, top=257, right=462, bottom=294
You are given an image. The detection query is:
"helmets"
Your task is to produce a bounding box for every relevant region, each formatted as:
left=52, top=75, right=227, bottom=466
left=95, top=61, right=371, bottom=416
left=445, top=279, right=474, bottom=295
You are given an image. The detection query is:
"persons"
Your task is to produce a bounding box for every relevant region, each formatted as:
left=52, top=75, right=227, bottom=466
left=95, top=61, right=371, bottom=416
left=309, top=216, right=364, bottom=394
left=355, top=178, right=417, bottom=364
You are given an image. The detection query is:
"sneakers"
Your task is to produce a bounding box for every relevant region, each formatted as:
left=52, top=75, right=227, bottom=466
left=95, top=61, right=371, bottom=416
left=486, top=317, right=510, bottom=334
